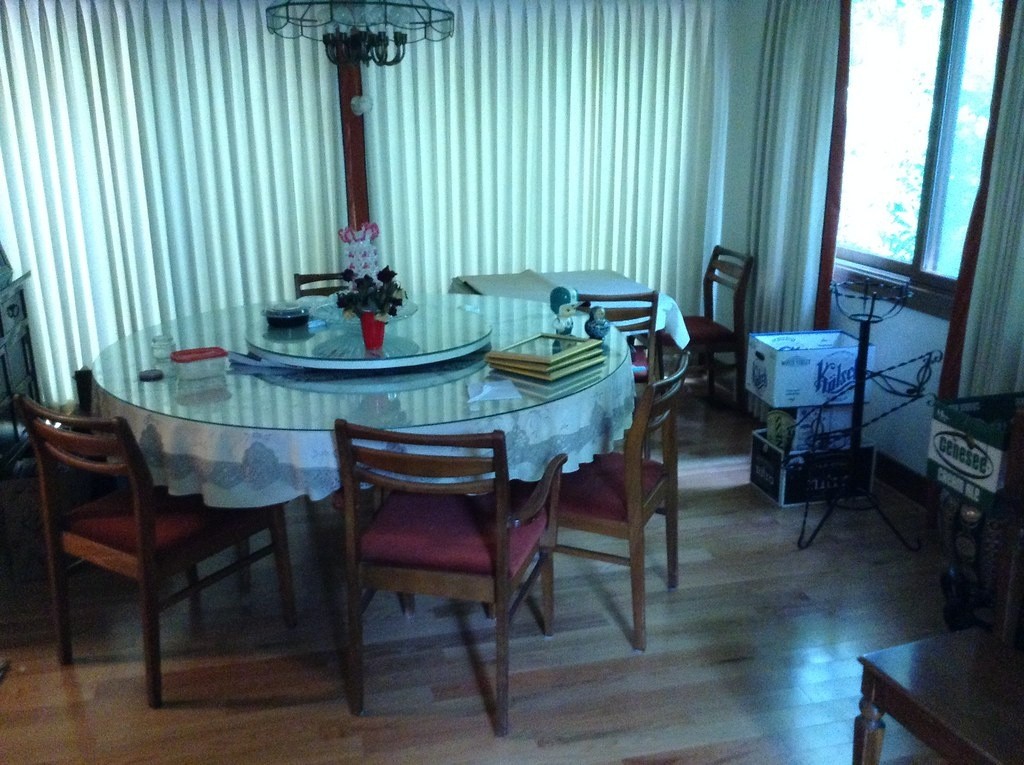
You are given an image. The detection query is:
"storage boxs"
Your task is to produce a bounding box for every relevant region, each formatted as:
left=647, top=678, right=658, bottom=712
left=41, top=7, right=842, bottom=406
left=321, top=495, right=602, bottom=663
left=744, top=327, right=876, bottom=409
left=789, top=407, right=853, bottom=451
left=925, top=389, right=1024, bottom=507
left=747, top=423, right=876, bottom=508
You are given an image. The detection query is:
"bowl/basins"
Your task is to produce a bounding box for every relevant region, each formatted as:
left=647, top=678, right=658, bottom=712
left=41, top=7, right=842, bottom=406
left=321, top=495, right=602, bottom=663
left=260, top=302, right=313, bottom=327
left=169, top=347, right=227, bottom=380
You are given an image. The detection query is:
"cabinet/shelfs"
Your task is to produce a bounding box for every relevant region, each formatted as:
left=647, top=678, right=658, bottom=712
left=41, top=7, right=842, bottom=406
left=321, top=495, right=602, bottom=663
left=0, top=270, right=64, bottom=575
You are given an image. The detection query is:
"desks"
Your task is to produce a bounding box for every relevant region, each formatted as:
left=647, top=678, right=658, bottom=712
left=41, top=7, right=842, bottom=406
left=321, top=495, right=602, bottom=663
left=451, top=269, right=691, bottom=357
left=79, top=291, right=635, bottom=634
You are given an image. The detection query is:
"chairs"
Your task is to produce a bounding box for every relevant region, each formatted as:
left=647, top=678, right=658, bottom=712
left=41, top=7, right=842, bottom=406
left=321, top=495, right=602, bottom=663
left=332, top=413, right=568, bottom=739
left=293, top=271, right=350, bottom=301
left=530, top=350, right=692, bottom=655
left=570, top=287, right=660, bottom=458
left=849, top=482, right=1023, bottom=765
left=14, top=389, right=295, bottom=710
left=660, top=245, right=760, bottom=415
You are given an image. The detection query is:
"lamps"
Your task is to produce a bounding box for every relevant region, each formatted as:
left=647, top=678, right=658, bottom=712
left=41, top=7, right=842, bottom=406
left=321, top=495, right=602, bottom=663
left=265, top=0, right=455, bottom=115
